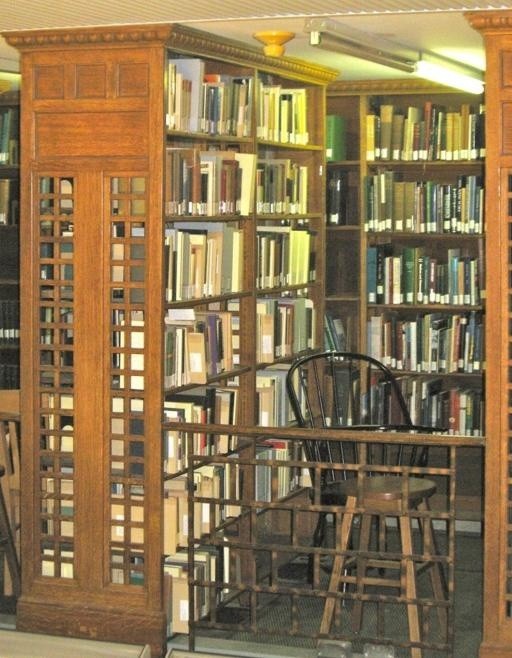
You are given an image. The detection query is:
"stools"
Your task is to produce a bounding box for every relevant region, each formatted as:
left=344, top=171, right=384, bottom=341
left=317, top=476, right=450, bottom=658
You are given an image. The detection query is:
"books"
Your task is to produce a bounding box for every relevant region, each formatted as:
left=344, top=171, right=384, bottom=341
left=324, top=169, right=358, bottom=222
left=0, top=107, right=20, bottom=165
left=114, top=306, right=234, bottom=395
left=366, top=103, right=484, bottom=162
left=0, top=178, right=19, bottom=226
left=255, top=225, right=319, bottom=290
left=367, top=311, right=485, bottom=374
left=107, top=462, right=238, bottom=559
left=379, top=377, right=482, bottom=431
left=254, top=299, right=318, bottom=368
left=41, top=177, right=80, bottom=579
left=0, top=284, right=22, bottom=350
left=116, top=140, right=257, bottom=215
left=106, top=560, right=231, bottom=635
left=110, top=383, right=238, bottom=475
left=369, top=242, right=485, bottom=306
left=324, top=114, right=346, bottom=162
left=326, top=238, right=359, bottom=297
left=257, top=81, right=310, bottom=145
left=0, top=223, right=20, bottom=281
left=324, top=368, right=361, bottom=430
left=259, top=157, right=311, bottom=217
left=164, top=58, right=253, bottom=135
left=364, top=171, right=485, bottom=234
left=255, top=363, right=316, bottom=431
left=324, top=311, right=354, bottom=358
left=114, top=228, right=245, bottom=303
left=255, top=439, right=314, bottom=502
left=0, top=346, right=21, bottom=391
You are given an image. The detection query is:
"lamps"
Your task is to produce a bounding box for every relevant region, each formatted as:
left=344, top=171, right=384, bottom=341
left=410, top=49, right=487, bottom=97
left=299, top=17, right=421, bottom=75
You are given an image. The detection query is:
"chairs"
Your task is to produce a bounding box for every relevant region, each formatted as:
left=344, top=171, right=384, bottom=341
left=286, top=351, right=449, bottom=599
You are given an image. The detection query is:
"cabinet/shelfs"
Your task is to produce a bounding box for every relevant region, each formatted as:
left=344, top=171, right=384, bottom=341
left=319, top=78, right=484, bottom=536
left=464, top=11, right=512, bottom=658
left=0, top=21, right=338, bottom=658
